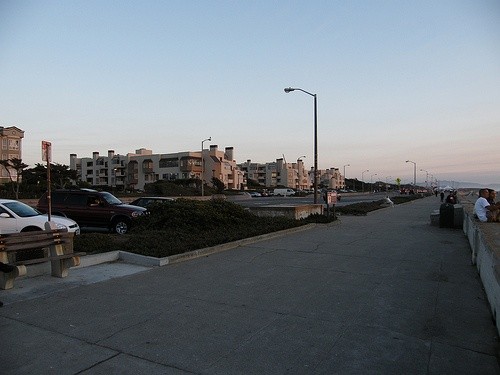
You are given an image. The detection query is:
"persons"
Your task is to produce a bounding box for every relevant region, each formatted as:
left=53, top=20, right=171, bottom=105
left=473, top=188, right=500, bottom=223
left=386, top=196, right=393, bottom=204
left=90, top=195, right=101, bottom=206
left=446, top=191, right=457, bottom=204
left=440, top=189, right=445, bottom=202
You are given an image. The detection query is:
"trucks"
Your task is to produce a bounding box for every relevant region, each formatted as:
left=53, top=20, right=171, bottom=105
left=275, top=188, right=295, bottom=197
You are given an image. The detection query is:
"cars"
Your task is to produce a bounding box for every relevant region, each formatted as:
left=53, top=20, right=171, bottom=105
left=251, top=191, right=274, bottom=197
left=0, top=199, right=80, bottom=259
left=399, top=188, right=428, bottom=195
left=127, top=197, right=175, bottom=212
left=294, top=188, right=356, bottom=201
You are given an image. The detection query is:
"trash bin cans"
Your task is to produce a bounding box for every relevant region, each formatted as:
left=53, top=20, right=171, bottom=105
left=440, top=203, right=451, bottom=227
left=446, top=204, right=464, bottom=228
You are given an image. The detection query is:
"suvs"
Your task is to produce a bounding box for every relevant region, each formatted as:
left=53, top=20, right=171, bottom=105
left=35, top=186, right=150, bottom=235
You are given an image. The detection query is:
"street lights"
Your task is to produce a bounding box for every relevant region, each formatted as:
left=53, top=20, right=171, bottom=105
left=362, top=170, right=369, bottom=193
left=284, top=87, right=318, bottom=204
left=406, top=161, right=416, bottom=190
left=201, top=139, right=211, bottom=196
left=371, top=174, right=377, bottom=192
left=420, top=169, right=428, bottom=185
left=344, top=164, right=350, bottom=193
left=297, top=156, right=306, bottom=195
left=113, top=167, right=118, bottom=185
left=431, top=176, right=437, bottom=186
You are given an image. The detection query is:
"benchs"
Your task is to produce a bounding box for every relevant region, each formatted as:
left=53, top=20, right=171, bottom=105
left=0, top=222, right=87, bottom=290
